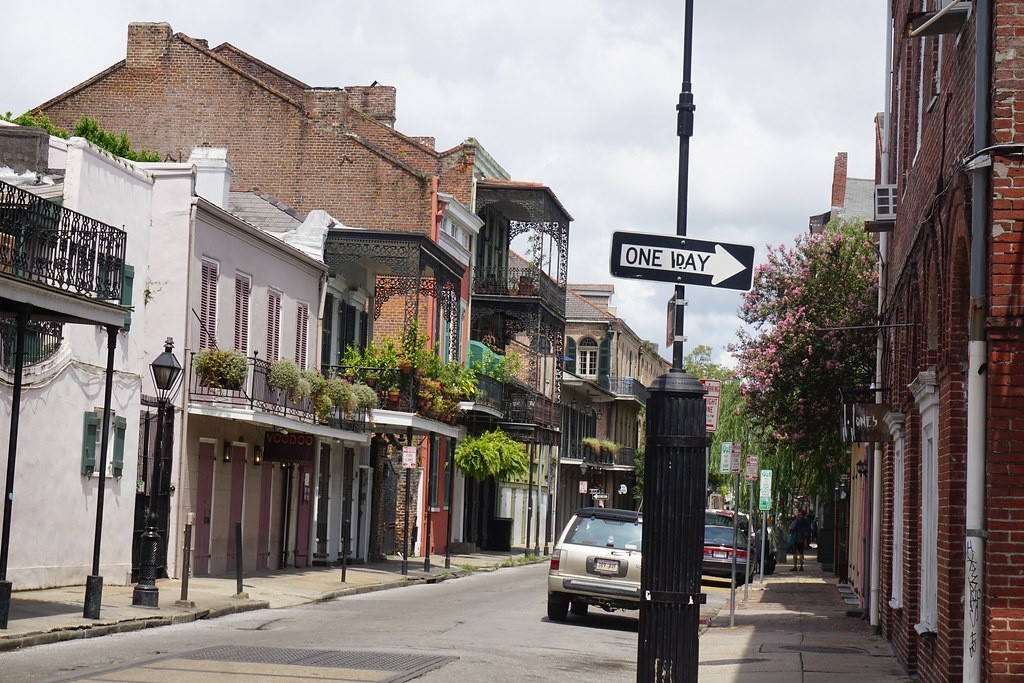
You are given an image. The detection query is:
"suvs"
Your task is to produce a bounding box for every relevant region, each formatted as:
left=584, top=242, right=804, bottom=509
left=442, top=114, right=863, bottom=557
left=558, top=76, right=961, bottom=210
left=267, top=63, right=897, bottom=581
left=545, top=508, right=642, bottom=622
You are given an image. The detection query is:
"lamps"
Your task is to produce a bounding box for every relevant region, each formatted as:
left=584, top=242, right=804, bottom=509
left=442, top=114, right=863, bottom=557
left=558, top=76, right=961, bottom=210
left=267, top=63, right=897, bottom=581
left=839, top=482, right=850, bottom=493
left=223, top=440, right=232, bottom=463
left=856, top=459, right=869, bottom=480
left=254, top=444, right=262, bottom=466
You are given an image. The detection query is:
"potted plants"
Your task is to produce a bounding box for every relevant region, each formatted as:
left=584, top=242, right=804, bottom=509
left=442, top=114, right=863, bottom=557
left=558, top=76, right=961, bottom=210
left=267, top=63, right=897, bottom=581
left=191, top=318, right=529, bottom=483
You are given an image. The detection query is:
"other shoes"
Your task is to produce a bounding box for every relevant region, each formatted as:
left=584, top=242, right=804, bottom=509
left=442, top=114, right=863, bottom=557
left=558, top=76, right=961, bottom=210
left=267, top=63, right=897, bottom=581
left=799, top=566, right=804, bottom=570
left=790, top=567, right=798, bottom=571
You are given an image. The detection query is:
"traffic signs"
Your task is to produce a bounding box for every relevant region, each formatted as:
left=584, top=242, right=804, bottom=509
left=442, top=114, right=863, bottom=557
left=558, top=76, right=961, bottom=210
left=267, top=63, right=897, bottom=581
left=611, top=233, right=754, bottom=292
left=592, top=493, right=609, bottom=500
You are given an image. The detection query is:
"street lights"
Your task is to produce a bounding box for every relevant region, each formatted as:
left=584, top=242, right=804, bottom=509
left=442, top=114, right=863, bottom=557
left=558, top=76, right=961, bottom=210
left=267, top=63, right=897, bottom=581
left=131, top=340, right=181, bottom=608
left=580, top=456, right=587, bottom=508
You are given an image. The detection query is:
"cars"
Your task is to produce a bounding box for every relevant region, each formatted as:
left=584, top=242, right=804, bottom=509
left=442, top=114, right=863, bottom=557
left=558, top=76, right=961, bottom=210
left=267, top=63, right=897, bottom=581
left=703, top=526, right=756, bottom=586
left=705, top=509, right=754, bottom=545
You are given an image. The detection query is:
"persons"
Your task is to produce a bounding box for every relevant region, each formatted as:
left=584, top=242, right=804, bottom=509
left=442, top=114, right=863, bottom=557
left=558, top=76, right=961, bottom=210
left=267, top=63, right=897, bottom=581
left=787, top=510, right=817, bottom=571
left=766, top=513, right=778, bottom=563
left=777, top=513, right=783, bottom=531
